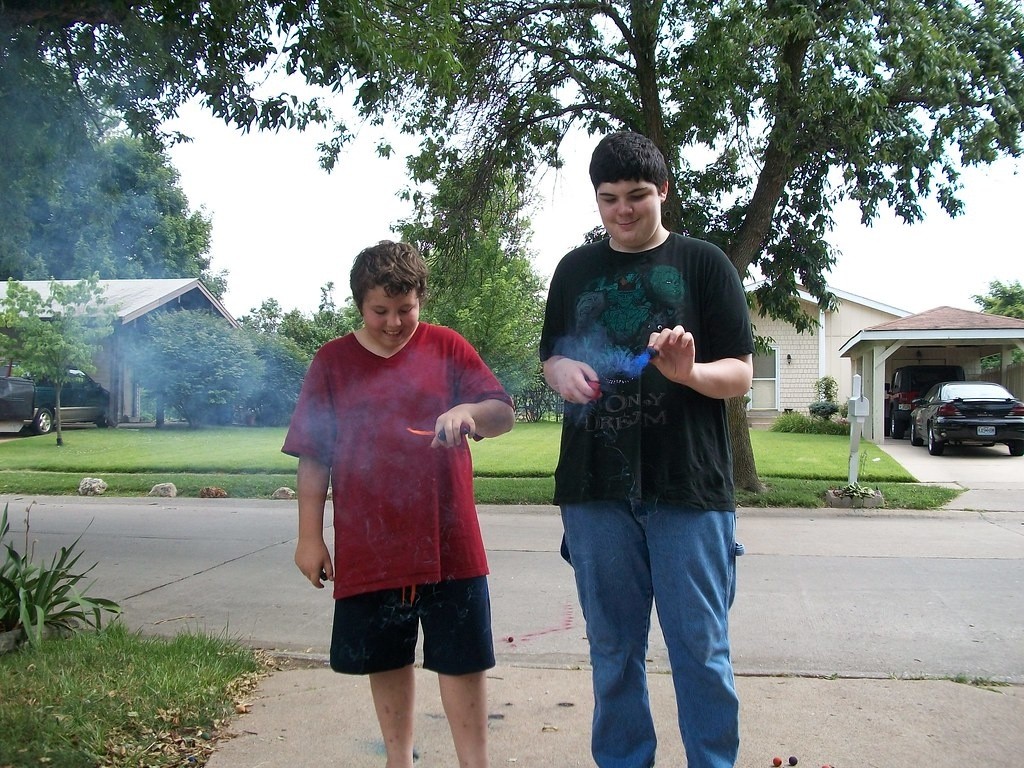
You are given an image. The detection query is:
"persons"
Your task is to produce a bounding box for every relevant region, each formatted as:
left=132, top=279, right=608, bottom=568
left=280, top=240, right=514, bottom=768
left=539, top=132, right=756, bottom=768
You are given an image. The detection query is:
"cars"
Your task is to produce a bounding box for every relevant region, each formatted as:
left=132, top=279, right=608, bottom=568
left=910, top=380, right=1024, bottom=457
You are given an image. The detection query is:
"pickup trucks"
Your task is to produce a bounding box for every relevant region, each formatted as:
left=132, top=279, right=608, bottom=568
left=0, top=361, right=111, bottom=435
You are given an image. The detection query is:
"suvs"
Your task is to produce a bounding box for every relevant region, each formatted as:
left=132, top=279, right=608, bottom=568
left=884, top=365, right=965, bottom=439
